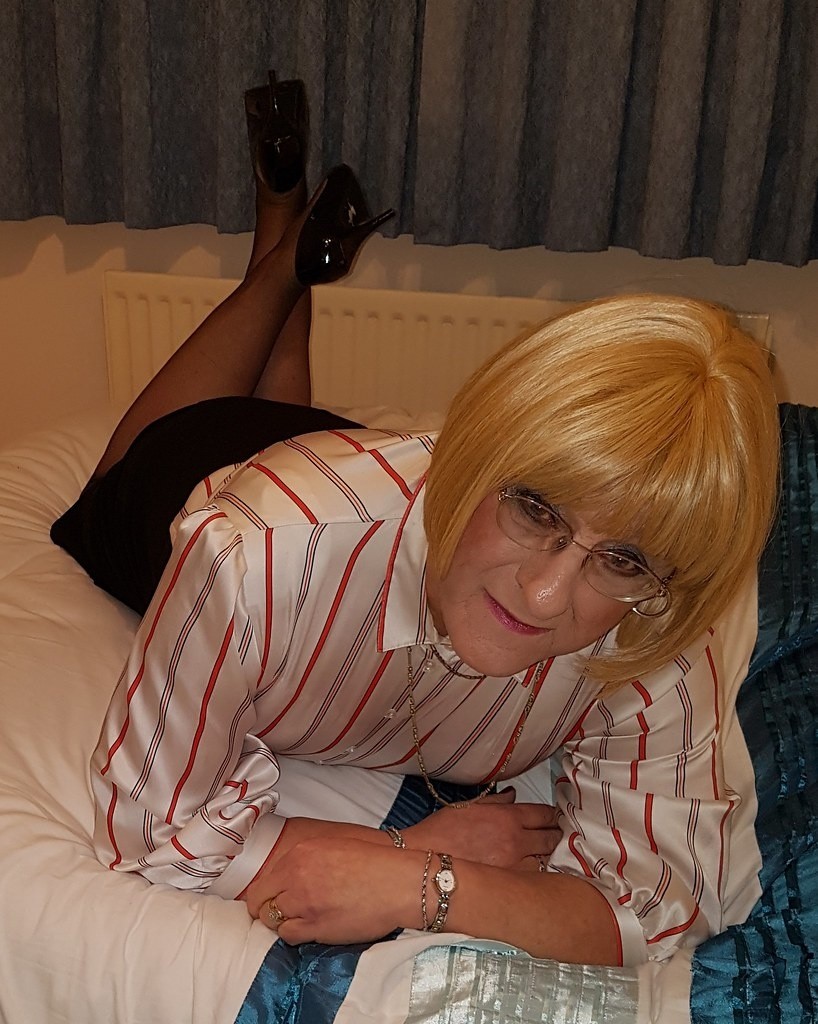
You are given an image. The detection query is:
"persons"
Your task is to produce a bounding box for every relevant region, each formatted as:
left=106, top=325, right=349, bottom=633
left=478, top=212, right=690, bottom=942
left=49, top=71, right=782, bottom=967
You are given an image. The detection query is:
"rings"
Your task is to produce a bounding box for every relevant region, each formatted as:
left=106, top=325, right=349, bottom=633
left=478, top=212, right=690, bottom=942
left=534, top=853, right=544, bottom=872
left=267, top=896, right=288, bottom=924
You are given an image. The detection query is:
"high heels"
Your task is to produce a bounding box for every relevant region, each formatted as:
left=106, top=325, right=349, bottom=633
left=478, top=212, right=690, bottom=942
left=244, top=67, right=303, bottom=193
left=295, top=164, right=395, bottom=286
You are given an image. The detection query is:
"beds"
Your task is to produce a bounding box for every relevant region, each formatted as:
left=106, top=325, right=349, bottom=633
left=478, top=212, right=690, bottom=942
left=0, top=401, right=818, bottom=1024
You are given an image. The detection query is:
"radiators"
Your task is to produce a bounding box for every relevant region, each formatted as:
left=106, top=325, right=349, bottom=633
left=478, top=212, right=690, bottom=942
left=104, top=270, right=774, bottom=404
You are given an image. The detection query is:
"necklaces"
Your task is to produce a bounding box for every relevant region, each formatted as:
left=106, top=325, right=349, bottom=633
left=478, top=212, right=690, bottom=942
left=406, top=641, right=545, bottom=810
left=429, top=644, right=488, bottom=679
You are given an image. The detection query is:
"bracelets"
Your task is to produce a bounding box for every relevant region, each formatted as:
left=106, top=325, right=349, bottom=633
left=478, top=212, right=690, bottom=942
left=422, top=849, right=433, bottom=931
left=386, top=823, right=405, bottom=849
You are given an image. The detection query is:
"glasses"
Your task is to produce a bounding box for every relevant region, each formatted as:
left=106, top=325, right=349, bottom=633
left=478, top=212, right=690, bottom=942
left=496, top=489, right=671, bottom=619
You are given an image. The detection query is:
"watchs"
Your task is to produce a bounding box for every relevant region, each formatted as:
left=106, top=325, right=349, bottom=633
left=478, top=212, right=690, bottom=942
left=428, top=853, right=457, bottom=933
